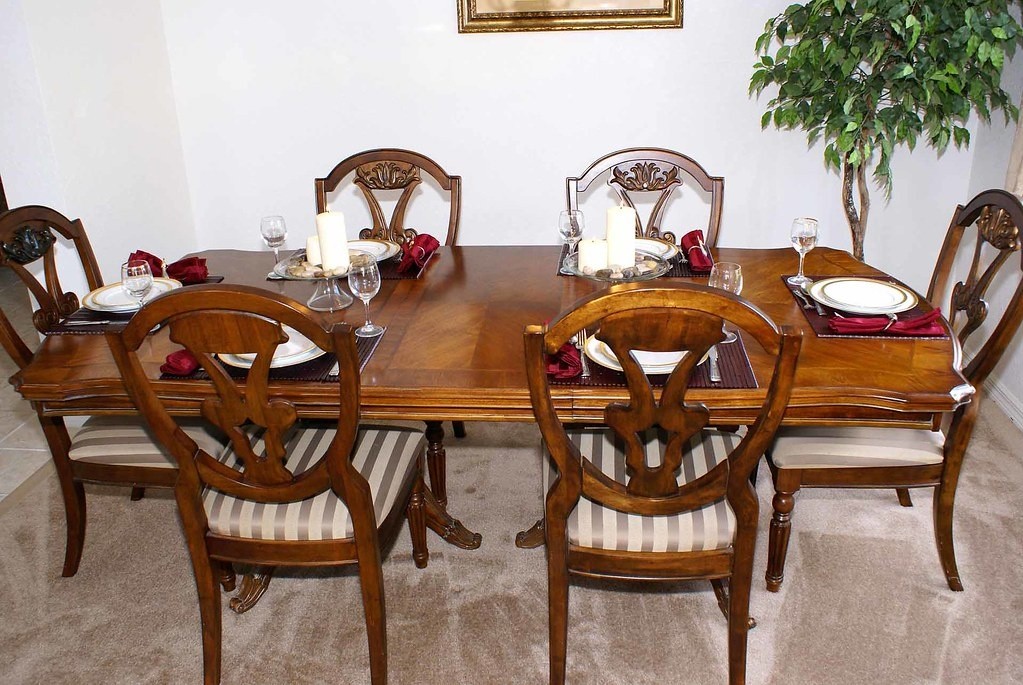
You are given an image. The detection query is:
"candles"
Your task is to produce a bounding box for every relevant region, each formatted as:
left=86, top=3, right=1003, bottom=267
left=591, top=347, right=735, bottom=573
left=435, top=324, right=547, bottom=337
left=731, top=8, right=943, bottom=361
left=579, top=236, right=608, bottom=272
left=316, top=204, right=351, bottom=268
left=606, top=198, right=637, bottom=269
left=306, top=235, right=322, bottom=266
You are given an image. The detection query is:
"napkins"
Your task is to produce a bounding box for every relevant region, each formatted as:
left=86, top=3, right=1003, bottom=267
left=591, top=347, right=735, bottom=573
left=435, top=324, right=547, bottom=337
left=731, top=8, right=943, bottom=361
left=159, top=348, right=201, bottom=377
left=681, top=228, right=714, bottom=272
left=126, top=249, right=210, bottom=286
left=541, top=316, right=584, bottom=380
left=825, top=307, right=946, bottom=335
left=396, top=232, right=440, bottom=273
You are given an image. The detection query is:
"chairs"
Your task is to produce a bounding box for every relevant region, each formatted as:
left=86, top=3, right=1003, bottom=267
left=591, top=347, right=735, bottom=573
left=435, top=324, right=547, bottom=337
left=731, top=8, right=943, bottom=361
left=566, top=146, right=725, bottom=248
left=764, top=189, right=1023, bottom=594
left=104, top=282, right=427, bottom=685
left=314, top=148, right=462, bottom=509
left=523, top=280, right=803, bottom=685
left=0, top=206, right=236, bottom=590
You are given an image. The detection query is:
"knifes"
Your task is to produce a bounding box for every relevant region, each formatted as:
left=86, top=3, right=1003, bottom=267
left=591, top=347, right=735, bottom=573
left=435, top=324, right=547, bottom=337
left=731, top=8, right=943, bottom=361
left=329, top=336, right=359, bottom=376
left=708, top=345, right=721, bottom=381
left=64, top=320, right=129, bottom=326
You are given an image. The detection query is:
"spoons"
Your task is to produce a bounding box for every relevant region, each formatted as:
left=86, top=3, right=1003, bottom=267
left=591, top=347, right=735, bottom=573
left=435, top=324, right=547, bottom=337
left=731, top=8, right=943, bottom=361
left=800, top=282, right=827, bottom=315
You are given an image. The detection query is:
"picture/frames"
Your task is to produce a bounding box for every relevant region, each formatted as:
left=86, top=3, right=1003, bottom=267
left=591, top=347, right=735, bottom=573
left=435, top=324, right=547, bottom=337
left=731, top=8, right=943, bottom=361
left=456, top=0, right=685, bottom=33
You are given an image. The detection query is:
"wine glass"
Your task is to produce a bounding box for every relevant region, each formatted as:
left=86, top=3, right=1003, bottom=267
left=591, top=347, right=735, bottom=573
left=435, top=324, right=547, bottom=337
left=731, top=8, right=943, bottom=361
left=259, top=215, right=286, bottom=265
left=122, top=260, right=160, bottom=331
left=787, top=218, right=818, bottom=286
left=557, top=210, right=584, bottom=275
left=707, top=262, right=743, bottom=344
left=348, top=255, right=384, bottom=337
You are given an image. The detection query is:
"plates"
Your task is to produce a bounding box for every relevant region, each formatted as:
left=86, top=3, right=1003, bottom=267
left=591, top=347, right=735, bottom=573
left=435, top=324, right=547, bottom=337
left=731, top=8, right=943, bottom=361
left=584, top=329, right=708, bottom=374
left=347, top=240, right=402, bottom=261
left=217, top=323, right=328, bottom=369
left=811, top=277, right=919, bottom=316
left=636, top=238, right=678, bottom=260
left=82, top=277, right=182, bottom=313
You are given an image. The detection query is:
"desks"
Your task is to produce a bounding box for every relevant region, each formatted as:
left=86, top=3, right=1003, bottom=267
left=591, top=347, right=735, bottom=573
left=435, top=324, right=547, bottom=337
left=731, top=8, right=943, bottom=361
left=9, top=249, right=976, bottom=612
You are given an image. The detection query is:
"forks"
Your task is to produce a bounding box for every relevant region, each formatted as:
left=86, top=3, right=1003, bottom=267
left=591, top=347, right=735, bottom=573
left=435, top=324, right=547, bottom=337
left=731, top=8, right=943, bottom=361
left=793, top=289, right=819, bottom=309
left=575, top=327, right=591, bottom=377
left=679, top=247, right=689, bottom=264
